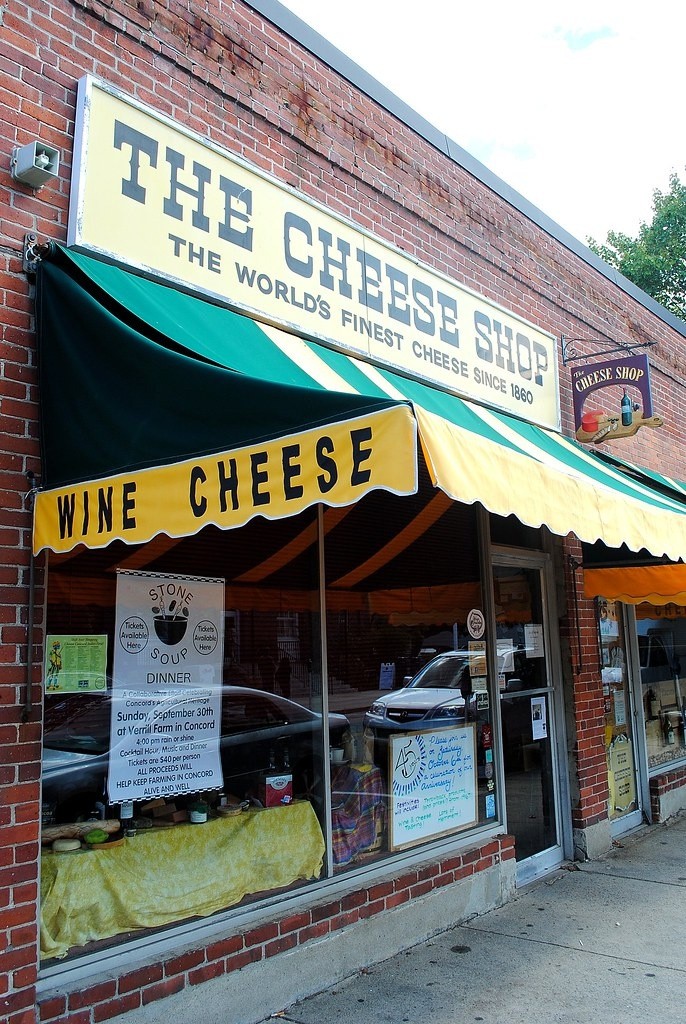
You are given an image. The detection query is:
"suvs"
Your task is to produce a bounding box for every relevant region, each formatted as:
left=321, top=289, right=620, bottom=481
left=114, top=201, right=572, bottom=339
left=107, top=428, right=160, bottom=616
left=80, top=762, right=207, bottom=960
left=364, top=644, right=549, bottom=771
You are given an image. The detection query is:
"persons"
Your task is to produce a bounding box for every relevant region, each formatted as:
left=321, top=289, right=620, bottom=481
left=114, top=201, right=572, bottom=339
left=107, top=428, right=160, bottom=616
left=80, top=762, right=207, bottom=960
left=258, top=651, right=277, bottom=692
left=275, top=657, right=293, bottom=698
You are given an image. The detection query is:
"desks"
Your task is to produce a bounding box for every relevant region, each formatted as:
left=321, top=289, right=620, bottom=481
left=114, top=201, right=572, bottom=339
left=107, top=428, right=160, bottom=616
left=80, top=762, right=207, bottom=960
left=41, top=800, right=327, bottom=961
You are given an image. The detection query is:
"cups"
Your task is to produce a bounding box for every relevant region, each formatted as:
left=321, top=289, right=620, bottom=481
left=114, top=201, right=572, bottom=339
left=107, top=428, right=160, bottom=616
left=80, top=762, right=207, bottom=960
left=330, top=748, right=344, bottom=761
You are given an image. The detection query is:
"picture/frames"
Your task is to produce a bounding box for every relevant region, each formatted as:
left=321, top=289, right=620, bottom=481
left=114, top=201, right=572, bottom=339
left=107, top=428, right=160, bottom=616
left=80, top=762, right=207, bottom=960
left=389, top=722, right=479, bottom=852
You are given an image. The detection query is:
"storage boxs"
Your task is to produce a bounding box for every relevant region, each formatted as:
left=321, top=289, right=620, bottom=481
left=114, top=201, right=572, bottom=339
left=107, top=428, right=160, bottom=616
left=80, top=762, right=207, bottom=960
left=258, top=773, right=293, bottom=808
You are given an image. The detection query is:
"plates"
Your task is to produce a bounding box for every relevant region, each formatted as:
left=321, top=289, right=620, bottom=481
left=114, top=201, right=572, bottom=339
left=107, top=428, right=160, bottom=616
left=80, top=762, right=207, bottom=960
left=329, top=759, right=348, bottom=765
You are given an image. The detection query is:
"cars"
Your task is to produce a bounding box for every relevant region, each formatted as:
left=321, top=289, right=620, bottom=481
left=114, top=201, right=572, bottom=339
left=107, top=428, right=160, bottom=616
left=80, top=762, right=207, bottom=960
left=42, top=686, right=351, bottom=826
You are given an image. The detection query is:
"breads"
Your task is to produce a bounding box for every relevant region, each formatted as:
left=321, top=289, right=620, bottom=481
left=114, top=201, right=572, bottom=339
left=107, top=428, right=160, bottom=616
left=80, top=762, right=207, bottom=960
left=41, top=818, right=120, bottom=843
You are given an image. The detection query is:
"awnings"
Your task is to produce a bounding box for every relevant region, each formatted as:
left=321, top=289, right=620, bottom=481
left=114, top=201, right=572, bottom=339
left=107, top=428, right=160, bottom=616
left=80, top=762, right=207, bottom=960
left=29, top=241, right=685, bottom=561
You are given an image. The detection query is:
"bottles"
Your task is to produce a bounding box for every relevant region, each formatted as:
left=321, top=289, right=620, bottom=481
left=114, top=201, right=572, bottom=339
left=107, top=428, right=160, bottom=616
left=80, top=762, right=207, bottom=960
left=191, top=793, right=208, bottom=825
left=664, top=713, right=675, bottom=744
left=281, top=748, right=291, bottom=773
left=647, top=686, right=660, bottom=719
left=117, top=802, right=134, bottom=828
left=217, top=789, right=228, bottom=806
left=269, top=750, right=278, bottom=774
